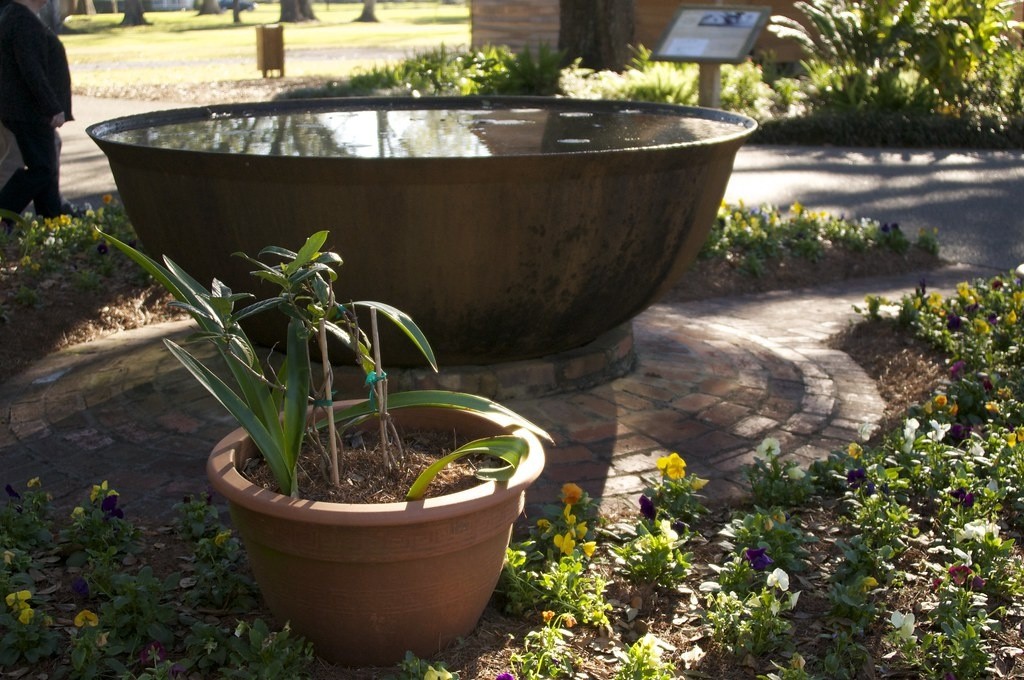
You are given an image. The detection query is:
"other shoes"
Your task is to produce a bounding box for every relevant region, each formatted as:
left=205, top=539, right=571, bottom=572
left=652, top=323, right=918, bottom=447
left=0, top=222, right=28, bottom=239
left=39, top=204, right=92, bottom=224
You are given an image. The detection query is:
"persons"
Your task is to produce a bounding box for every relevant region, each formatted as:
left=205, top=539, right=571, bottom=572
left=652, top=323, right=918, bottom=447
left=0, top=0, right=88, bottom=235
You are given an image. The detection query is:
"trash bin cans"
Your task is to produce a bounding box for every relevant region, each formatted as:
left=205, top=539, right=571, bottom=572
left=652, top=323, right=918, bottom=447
left=255, top=24, right=284, bottom=79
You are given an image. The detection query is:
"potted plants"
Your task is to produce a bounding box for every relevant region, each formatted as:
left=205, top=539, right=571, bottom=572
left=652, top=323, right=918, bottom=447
left=87, top=221, right=558, bottom=669
